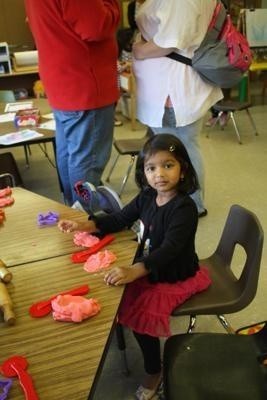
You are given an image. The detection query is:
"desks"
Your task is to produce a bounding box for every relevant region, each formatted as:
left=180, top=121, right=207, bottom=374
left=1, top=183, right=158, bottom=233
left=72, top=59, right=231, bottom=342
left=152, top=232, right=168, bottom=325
left=117, top=60, right=138, bottom=130
left=0, top=188, right=138, bottom=400
left=0, top=101, right=63, bottom=195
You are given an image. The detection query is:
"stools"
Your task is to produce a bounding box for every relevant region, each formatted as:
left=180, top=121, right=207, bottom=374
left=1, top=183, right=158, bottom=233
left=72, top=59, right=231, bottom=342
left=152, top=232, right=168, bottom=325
left=205, top=100, right=259, bottom=145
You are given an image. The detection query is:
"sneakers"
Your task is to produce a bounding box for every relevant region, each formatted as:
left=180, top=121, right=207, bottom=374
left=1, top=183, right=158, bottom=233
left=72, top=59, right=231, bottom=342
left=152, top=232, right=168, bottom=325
left=205, top=113, right=227, bottom=126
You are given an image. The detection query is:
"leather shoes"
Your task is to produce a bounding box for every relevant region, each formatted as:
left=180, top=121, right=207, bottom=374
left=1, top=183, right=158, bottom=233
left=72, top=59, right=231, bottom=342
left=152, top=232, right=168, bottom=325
left=135, top=366, right=163, bottom=400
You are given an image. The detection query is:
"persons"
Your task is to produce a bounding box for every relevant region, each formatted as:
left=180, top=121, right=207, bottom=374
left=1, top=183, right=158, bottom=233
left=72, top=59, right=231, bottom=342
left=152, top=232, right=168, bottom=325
left=131, top=0, right=223, bottom=216
left=56, top=132, right=213, bottom=400
left=23, top=0, right=122, bottom=217
left=204, top=106, right=229, bottom=128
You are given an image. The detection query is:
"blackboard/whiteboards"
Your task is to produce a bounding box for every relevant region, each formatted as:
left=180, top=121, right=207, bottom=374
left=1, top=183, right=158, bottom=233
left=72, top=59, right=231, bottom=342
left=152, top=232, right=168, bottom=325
left=245, top=8, right=267, bottom=47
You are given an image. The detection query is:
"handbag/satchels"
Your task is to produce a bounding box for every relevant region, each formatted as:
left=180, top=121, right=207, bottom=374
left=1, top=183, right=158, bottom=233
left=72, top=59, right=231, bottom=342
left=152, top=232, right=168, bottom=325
left=189, top=0, right=253, bottom=88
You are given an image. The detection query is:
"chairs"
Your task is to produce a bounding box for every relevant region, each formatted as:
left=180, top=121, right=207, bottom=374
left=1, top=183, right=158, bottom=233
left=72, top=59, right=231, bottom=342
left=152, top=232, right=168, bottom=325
left=105, top=126, right=157, bottom=197
left=111, top=203, right=267, bottom=400
